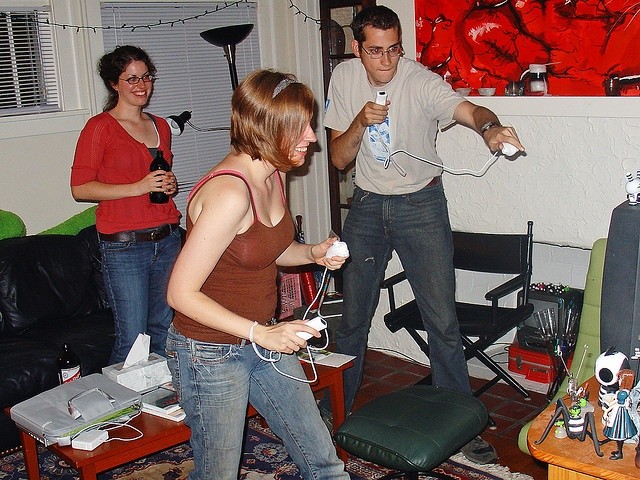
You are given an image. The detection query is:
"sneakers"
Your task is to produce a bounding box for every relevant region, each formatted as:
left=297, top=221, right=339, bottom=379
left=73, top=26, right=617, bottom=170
left=460, top=435, right=497, bottom=464
left=320, top=410, right=334, bottom=434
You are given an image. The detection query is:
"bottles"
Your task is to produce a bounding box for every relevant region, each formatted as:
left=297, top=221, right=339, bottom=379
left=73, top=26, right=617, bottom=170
left=149, top=150, right=170, bottom=204
left=57, top=343, right=81, bottom=385
left=528, top=63, right=547, bottom=92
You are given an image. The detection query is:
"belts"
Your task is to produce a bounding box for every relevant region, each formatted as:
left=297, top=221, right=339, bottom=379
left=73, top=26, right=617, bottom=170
left=99, top=224, right=178, bottom=243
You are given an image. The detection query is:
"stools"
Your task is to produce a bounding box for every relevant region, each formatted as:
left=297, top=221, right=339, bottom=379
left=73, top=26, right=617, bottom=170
left=333, top=385, right=489, bottom=479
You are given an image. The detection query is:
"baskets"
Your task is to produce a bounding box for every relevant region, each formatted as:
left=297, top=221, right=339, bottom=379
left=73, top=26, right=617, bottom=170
left=279, top=273, right=302, bottom=320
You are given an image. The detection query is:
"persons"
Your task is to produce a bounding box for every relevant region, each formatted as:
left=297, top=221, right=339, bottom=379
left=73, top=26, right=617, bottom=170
left=167, top=70, right=353, bottom=479
left=625, top=377, right=640, bottom=470
left=70, top=44, right=181, bottom=367
left=321, top=5, right=525, bottom=466
left=601, top=368, right=637, bottom=460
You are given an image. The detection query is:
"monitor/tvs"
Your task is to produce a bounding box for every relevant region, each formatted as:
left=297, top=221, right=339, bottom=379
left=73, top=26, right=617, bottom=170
left=517, top=285, right=584, bottom=353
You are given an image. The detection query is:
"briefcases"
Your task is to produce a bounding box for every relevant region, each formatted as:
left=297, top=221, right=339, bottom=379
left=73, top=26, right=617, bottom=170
left=508, top=342, right=574, bottom=383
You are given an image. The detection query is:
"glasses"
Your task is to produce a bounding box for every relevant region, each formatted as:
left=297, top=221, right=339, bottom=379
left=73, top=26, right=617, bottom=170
left=119, top=74, right=153, bottom=84
left=361, top=44, right=403, bottom=59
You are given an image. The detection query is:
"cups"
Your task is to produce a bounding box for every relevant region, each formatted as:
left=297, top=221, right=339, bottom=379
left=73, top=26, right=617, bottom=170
left=532, top=308, right=557, bottom=342
left=604, top=79, right=623, bottom=96
left=560, top=309, right=581, bottom=341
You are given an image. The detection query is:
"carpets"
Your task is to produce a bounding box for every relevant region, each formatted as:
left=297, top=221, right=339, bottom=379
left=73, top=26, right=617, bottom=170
left=0, top=415, right=534, bottom=480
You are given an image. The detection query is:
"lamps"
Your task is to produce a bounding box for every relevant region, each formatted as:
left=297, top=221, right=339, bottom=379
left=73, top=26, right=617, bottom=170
left=200, top=23, right=255, bottom=90
left=170, top=109, right=229, bottom=135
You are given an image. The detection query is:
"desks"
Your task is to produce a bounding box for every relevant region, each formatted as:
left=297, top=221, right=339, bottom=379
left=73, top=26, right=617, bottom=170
left=527, top=378, right=640, bottom=480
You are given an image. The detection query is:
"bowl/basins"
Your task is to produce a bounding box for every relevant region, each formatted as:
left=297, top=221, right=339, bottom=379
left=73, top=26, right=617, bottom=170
left=478, top=87, right=497, bottom=97
left=456, top=87, right=472, bottom=97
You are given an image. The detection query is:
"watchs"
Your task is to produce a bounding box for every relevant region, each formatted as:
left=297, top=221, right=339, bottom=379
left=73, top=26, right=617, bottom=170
left=479, top=120, right=503, bottom=139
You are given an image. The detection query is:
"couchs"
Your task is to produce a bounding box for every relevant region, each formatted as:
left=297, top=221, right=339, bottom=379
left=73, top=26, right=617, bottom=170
left=2, top=223, right=191, bottom=436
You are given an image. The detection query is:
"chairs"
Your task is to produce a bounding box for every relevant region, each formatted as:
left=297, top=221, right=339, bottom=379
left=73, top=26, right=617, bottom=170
left=380, top=220, right=536, bottom=431
left=515, top=237, right=611, bottom=455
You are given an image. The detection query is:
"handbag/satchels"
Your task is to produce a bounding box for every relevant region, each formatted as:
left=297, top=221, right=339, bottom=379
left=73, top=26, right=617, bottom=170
left=10, top=373, right=144, bottom=448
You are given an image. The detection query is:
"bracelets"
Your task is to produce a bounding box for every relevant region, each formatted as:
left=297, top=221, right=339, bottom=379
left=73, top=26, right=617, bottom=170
left=249, top=320, right=259, bottom=343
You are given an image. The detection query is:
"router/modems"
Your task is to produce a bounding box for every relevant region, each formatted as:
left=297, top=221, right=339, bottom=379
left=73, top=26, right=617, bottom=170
left=72, top=430, right=109, bottom=452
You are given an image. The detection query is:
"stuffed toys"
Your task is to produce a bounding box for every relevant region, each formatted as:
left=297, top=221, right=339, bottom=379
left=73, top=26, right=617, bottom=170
left=594, top=344, right=630, bottom=411
